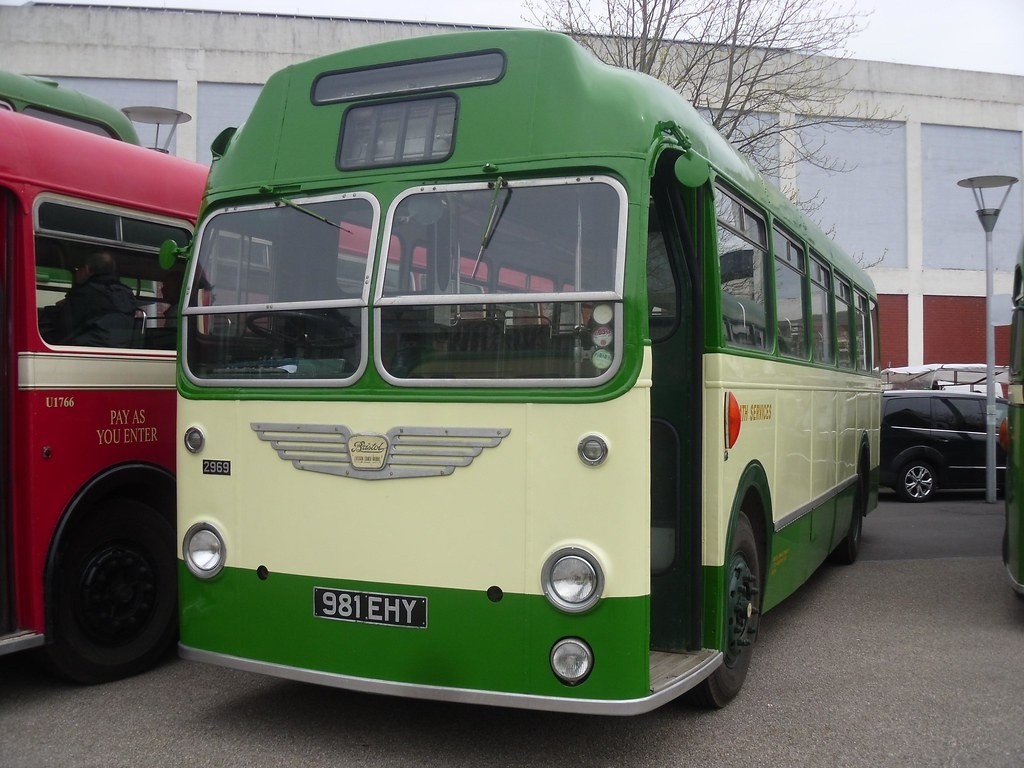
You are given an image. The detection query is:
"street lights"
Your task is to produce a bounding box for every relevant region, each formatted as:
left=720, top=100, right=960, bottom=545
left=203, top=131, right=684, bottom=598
left=122, top=107, right=194, bottom=157
left=957, top=175, right=1020, bottom=502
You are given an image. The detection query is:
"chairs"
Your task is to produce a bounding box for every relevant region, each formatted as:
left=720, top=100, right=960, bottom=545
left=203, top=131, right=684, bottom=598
left=131, top=308, right=232, bottom=353
left=722, top=301, right=864, bottom=370
left=382, top=308, right=584, bottom=381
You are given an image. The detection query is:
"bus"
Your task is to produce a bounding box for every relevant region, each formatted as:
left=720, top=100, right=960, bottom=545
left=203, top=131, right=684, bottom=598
left=160, top=28, right=885, bottom=716
left=1002, top=237, right=1024, bottom=596
left=0, top=69, right=144, bottom=146
left=0, top=108, right=592, bottom=685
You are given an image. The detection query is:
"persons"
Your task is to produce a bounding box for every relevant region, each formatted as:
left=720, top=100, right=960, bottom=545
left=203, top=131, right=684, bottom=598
left=36, top=249, right=144, bottom=348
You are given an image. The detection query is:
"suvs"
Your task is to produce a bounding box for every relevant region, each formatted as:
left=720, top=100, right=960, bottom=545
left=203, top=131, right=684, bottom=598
left=879, top=390, right=1010, bottom=503
